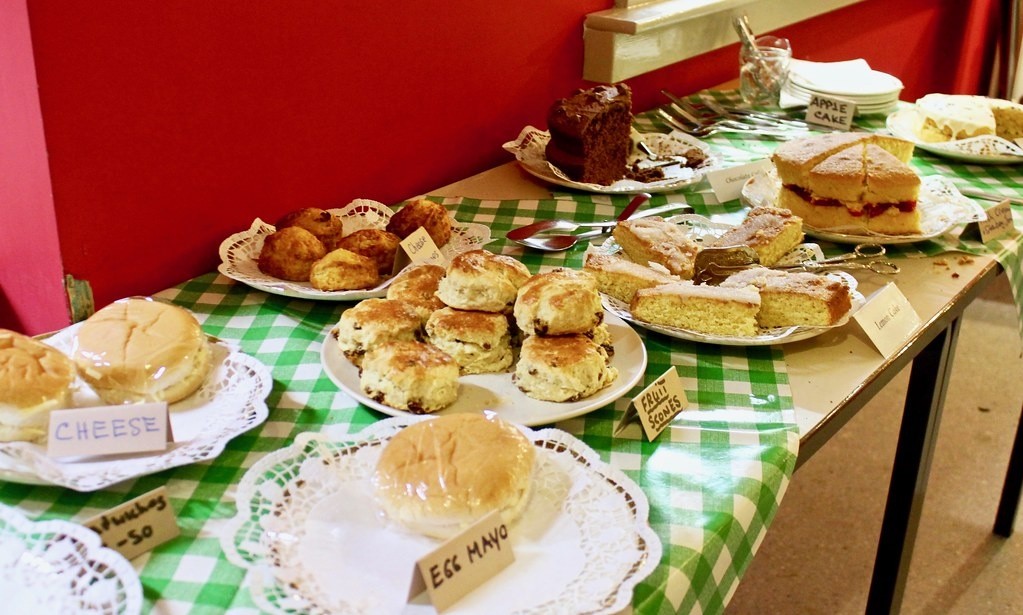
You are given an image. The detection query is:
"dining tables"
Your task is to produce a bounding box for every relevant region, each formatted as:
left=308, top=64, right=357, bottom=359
left=0, top=78, right=1023, bottom=615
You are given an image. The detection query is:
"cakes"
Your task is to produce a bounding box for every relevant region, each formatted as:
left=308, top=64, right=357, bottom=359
left=912, top=91, right=1023, bottom=147
left=543, top=84, right=633, bottom=186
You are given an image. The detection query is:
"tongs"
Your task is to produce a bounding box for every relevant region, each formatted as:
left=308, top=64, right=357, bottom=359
left=506, top=202, right=697, bottom=252
left=689, top=242, right=905, bottom=286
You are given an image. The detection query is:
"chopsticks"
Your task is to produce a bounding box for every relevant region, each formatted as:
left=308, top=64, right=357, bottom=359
left=885, top=103, right=1023, bottom=164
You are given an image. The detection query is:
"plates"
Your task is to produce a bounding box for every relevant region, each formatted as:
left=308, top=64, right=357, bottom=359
left=1, top=311, right=274, bottom=473
left=219, top=206, right=494, bottom=303
left=741, top=155, right=976, bottom=243
left=785, top=59, right=905, bottom=117
left=0, top=503, right=144, bottom=615
left=319, top=296, right=647, bottom=431
left=584, top=214, right=865, bottom=346
left=515, top=126, right=716, bottom=195
left=263, top=420, right=661, bottom=615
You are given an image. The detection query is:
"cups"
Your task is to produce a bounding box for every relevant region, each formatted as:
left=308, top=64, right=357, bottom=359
left=740, top=40, right=793, bottom=112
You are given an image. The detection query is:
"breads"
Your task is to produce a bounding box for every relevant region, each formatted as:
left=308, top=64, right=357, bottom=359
left=257, top=132, right=926, bottom=414
left=0, top=296, right=209, bottom=444
left=374, top=412, right=535, bottom=540
